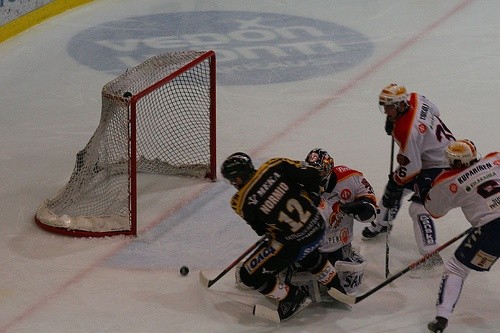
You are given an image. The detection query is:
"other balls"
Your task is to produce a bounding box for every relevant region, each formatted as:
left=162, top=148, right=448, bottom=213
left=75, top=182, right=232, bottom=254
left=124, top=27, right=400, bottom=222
left=180, top=266, right=189, bottom=276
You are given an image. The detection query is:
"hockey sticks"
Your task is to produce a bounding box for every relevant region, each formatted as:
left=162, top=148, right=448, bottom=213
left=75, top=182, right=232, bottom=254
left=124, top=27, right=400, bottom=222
left=327, top=227, right=475, bottom=305
left=384, top=136, right=396, bottom=285
left=199, top=235, right=267, bottom=288
left=252, top=304, right=280, bottom=322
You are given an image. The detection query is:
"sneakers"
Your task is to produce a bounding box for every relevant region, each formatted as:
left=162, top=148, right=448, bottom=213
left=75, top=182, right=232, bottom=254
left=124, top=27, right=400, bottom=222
left=409, top=253, right=445, bottom=278
left=277, top=281, right=312, bottom=320
left=334, top=284, right=352, bottom=310
left=361, top=221, right=392, bottom=240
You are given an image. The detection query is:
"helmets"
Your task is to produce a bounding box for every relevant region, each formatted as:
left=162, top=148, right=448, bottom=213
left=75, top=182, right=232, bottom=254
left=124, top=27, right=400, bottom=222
left=305, top=147, right=334, bottom=187
left=379, top=83, right=411, bottom=111
left=445, top=138, right=477, bottom=166
left=221, top=152, right=254, bottom=189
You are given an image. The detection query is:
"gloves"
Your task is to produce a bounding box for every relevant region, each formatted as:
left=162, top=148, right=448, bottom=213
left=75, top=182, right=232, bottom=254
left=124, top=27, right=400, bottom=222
left=382, top=173, right=403, bottom=208
left=413, top=173, right=433, bottom=199
left=385, top=118, right=394, bottom=135
left=339, top=200, right=367, bottom=220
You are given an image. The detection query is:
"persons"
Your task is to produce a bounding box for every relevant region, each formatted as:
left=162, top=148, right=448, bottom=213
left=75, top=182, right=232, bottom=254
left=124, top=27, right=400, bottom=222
left=362, top=84, right=457, bottom=277
left=219, top=152, right=348, bottom=320
left=417, top=138, right=500, bottom=333
left=235, top=148, right=381, bottom=303
